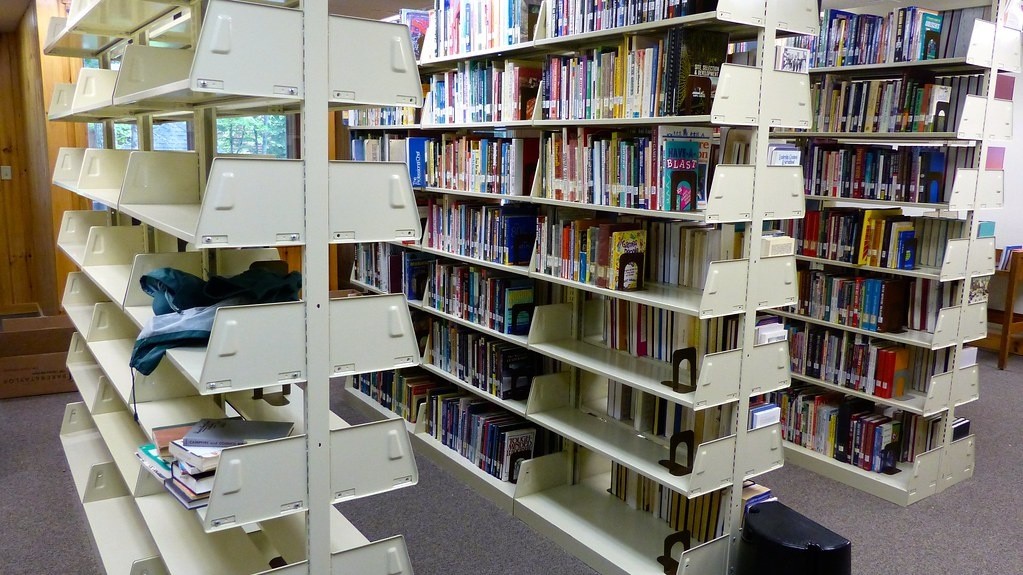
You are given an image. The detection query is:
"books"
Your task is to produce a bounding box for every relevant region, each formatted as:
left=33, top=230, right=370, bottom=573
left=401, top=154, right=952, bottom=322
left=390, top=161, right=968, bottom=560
left=344, top=0, right=727, bottom=127
left=607, top=377, right=971, bottom=546
left=722, top=9, right=995, bottom=71
left=131, top=418, right=298, bottom=513
left=348, top=130, right=721, bottom=290
left=768, top=77, right=993, bottom=136
left=602, top=214, right=1023, bottom=293
left=344, top=237, right=549, bottom=486
left=535, top=276, right=990, bottom=403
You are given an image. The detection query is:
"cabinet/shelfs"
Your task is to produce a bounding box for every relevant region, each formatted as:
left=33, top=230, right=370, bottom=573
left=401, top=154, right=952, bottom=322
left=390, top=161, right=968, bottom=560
left=782, top=0, right=1023, bottom=508
left=43, top=1, right=438, bottom=575
left=342, top=0, right=822, bottom=574
